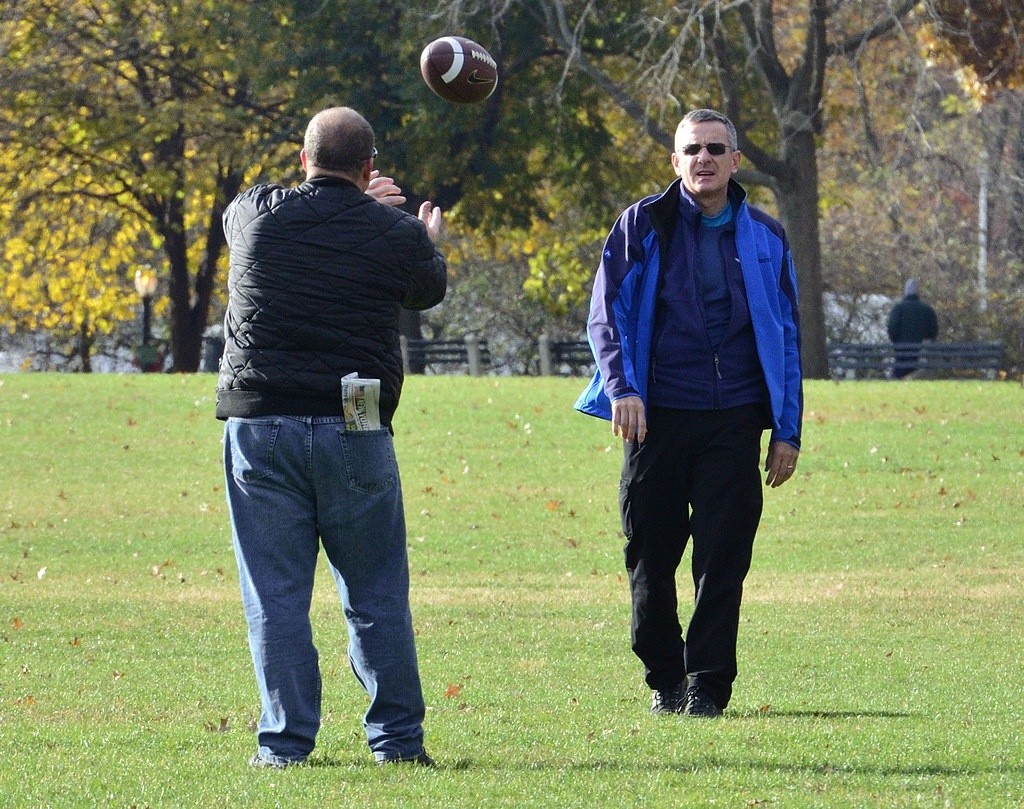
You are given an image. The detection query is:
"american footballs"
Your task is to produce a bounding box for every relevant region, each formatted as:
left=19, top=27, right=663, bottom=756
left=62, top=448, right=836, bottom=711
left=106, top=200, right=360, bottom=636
left=420, top=35, right=499, bottom=105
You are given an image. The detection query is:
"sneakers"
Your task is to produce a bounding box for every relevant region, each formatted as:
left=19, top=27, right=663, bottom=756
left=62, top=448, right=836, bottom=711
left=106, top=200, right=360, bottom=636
left=650, top=677, right=688, bottom=720
left=675, top=687, right=724, bottom=719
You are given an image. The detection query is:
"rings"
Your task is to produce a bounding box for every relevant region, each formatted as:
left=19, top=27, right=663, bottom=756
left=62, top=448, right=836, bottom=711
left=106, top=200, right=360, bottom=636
left=787, top=465, right=793, bottom=469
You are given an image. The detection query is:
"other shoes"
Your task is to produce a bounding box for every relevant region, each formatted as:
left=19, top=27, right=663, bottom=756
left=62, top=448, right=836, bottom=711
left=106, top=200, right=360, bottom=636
left=250, top=757, right=282, bottom=770
left=394, top=749, right=436, bottom=768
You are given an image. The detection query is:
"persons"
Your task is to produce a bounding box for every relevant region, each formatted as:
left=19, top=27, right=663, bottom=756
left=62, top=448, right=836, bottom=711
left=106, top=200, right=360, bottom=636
left=575, top=109, right=804, bottom=717
left=887, top=279, right=938, bottom=379
left=214, top=107, right=448, bottom=770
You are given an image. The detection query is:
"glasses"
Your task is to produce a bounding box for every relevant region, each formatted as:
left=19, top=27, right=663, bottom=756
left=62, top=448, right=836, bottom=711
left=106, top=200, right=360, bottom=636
left=363, top=147, right=378, bottom=159
left=676, top=143, right=736, bottom=156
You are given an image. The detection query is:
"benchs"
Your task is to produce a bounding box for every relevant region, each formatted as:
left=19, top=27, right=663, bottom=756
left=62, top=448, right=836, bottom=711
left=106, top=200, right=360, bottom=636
left=406, top=339, right=491, bottom=373
left=826, top=342, right=1002, bottom=379
left=552, top=341, right=596, bottom=375
